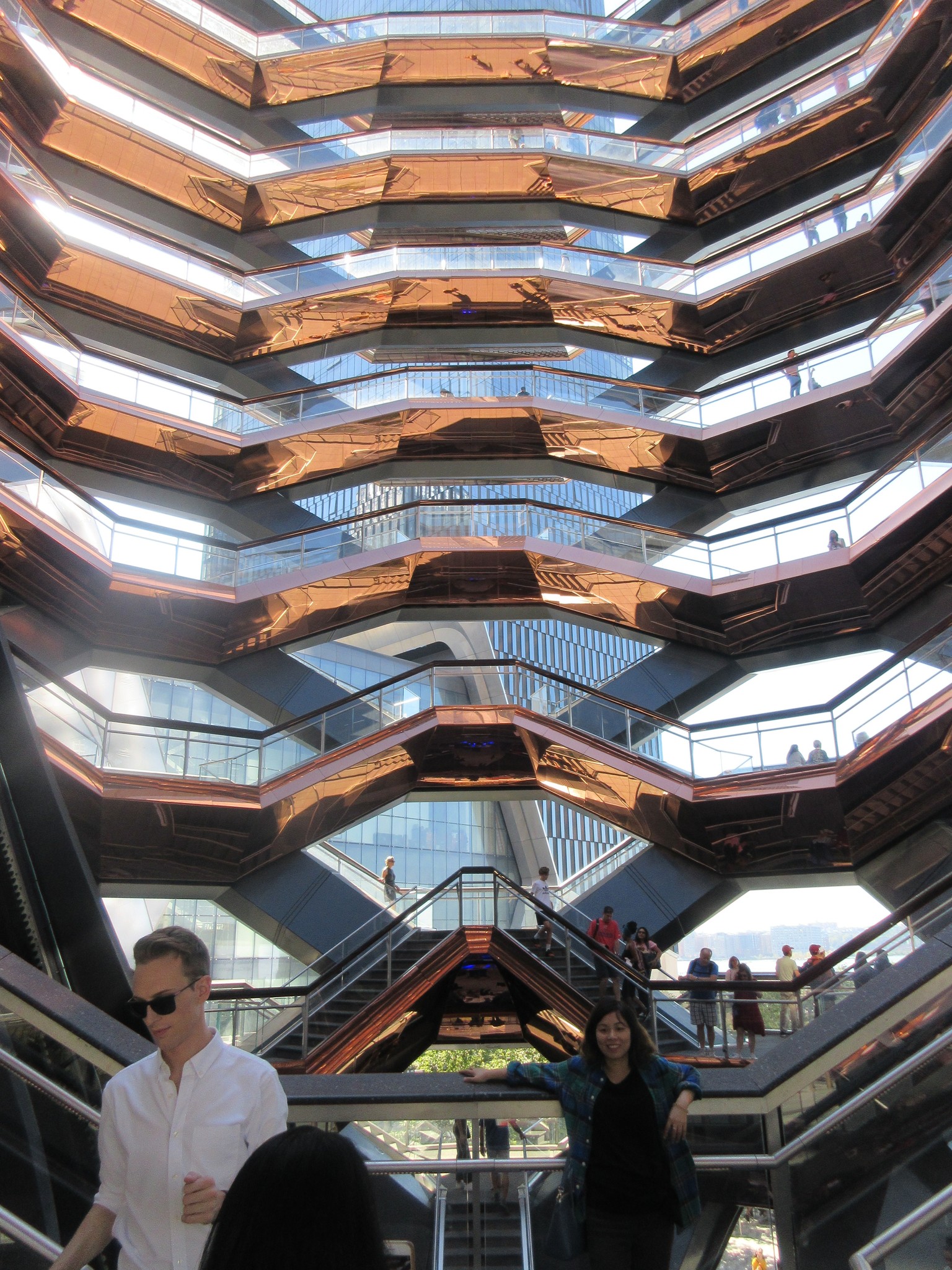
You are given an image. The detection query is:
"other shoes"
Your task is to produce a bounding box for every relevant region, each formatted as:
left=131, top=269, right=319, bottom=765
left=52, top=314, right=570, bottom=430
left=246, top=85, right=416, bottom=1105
left=456, top=1181, right=472, bottom=1192
left=729, top=1053, right=743, bottom=1059
left=545, top=949, right=555, bottom=957
left=708, top=1051, right=715, bottom=1057
left=699, top=1049, right=706, bottom=1057
left=532, top=935, right=541, bottom=947
left=750, top=1053, right=758, bottom=1060
left=779, top=1031, right=791, bottom=1037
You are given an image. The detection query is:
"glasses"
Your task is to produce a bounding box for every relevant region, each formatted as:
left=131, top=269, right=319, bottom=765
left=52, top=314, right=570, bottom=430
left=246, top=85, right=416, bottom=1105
left=388, top=860, right=395, bottom=863
left=127, top=979, right=203, bottom=1018
left=638, top=931, right=645, bottom=934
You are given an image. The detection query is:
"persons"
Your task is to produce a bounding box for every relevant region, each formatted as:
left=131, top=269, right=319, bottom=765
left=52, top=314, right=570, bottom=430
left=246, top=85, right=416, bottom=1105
left=679, top=950, right=718, bottom=1059
left=459, top=998, right=700, bottom=1270
left=779, top=943, right=893, bottom=1038
left=914, top=274, right=952, bottom=318
left=640, top=265, right=653, bottom=288
left=828, top=529, right=846, bottom=552
left=725, top=956, right=766, bottom=1060
left=199, top=1125, right=399, bottom=1270
left=588, top=906, right=661, bottom=1022
left=656, top=0, right=749, bottom=49
left=439, top=383, right=531, bottom=398
left=560, top=252, right=572, bottom=272
left=742, top=1203, right=768, bottom=1270
left=49, top=925, right=288, bottom=1270
left=377, top=857, right=401, bottom=913
left=786, top=733, right=868, bottom=767
left=531, top=867, right=556, bottom=956
left=508, top=116, right=587, bottom=155
left=779, top=348, right=820, bottom=397
left=755, top=7, right=908, bottom=134
left=801, top=161, right=905, bottom=249
left=453, top=1117, right=527, bottom=1200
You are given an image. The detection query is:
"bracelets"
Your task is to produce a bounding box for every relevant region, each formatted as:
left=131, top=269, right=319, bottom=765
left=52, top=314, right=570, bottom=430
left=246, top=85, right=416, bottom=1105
left=696, top=977, right=698, bottom=980
left=480, top=1142, right=484, bottom=1146
left=654, top=958, right=659, bottom=963
left=672, top=1103, right=689, bottom=1113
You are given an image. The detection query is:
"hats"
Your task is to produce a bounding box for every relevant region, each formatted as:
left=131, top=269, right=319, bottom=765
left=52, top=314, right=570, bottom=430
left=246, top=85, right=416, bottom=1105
left=809, top=945, right=821, bottom=955
left=782, top=945, right=794, bottom=953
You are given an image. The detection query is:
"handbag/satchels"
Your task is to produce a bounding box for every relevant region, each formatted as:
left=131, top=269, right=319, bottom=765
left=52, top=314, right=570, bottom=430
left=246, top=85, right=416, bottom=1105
left=642, top=952, right=662, bottom=969
